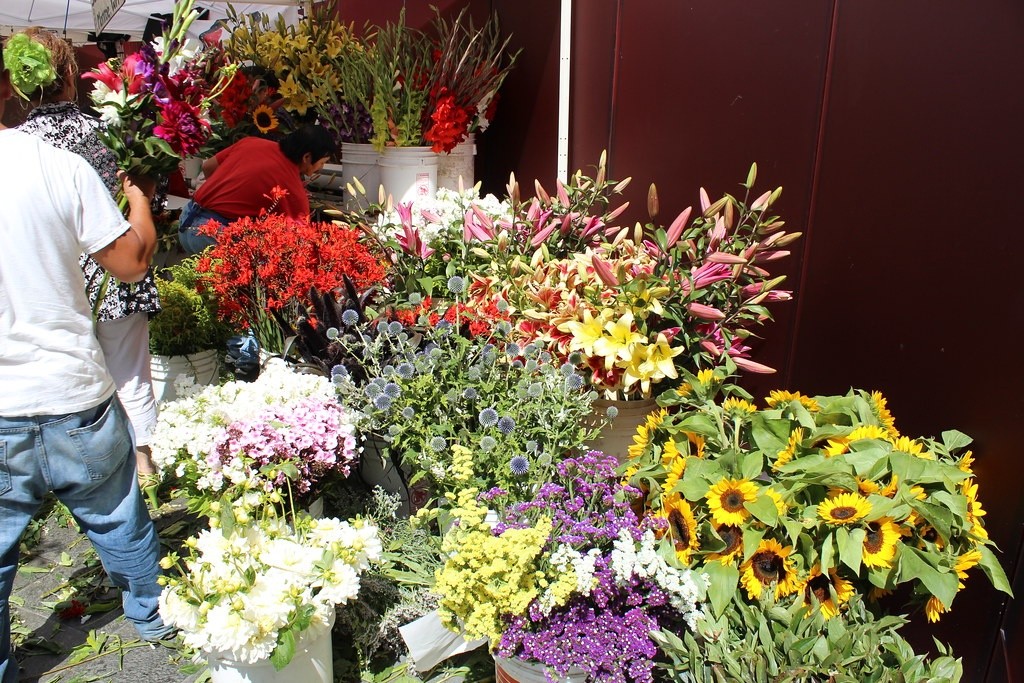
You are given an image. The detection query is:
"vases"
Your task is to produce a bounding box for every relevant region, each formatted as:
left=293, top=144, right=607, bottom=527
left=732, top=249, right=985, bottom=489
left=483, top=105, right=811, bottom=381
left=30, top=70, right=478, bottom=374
left=379, top=146, right=438, bottom=208
left=357, top=432, right=415, bottom=520
left=180, top=155, right=205, bottom=181
left=438, top=134, right=476, bottom=193
left=208, top=606, right=335, bottom=683
left=259, top=349, right=326, bottom=380
left=150, top=349, right=219, bottom=418
left=488, top=636, right=592, bottom=683
left=565, top=398, right=659, bottom=467
left=339, top=141, right=380, bottom=213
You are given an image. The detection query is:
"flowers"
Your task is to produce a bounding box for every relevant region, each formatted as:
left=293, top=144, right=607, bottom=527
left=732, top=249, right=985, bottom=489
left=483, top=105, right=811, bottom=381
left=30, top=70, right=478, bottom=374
left=82, top=0, right=1016, bottom=683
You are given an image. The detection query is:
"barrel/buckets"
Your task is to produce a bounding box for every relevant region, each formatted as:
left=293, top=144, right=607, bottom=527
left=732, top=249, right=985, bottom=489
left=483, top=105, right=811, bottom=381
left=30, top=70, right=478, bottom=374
left=150, top=348, right=220, bottom=404
left=496, top=651, right=588, bottom=683
left=338, top=133, right=478, bottom=215
left=574, top=397, right=663, bottom=462
left=362, top=437, right=436, bottom=520
left=197, top=607, right=338, bottom=683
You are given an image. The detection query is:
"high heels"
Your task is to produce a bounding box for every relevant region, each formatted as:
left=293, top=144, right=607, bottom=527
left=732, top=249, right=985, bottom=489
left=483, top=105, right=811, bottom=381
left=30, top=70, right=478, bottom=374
left=137, top=472, right=161, bottom=511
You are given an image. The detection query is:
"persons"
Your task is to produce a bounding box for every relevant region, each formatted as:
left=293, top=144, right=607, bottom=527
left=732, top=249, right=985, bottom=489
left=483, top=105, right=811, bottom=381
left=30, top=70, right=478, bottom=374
left=0, top=37, right=182, bottom=683
left=3, top=27, right=163, bottom=510
left=180, top=124, right=336, bottom=380
